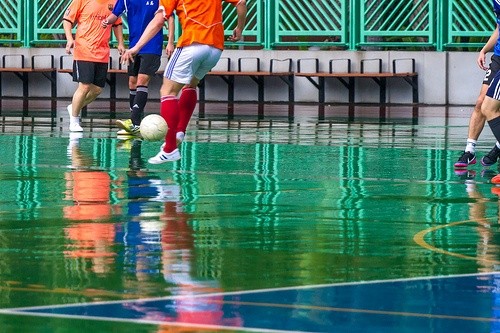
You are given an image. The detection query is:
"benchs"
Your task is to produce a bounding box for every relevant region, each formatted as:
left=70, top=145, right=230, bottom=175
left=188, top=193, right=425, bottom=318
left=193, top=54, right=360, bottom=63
left=1, top=54, right=57, bottom=98
left=294, top=56, right=420, bottom=104
left=57, top=54, right=164, bottom=99
left=196, top=56, right=294, bottom=102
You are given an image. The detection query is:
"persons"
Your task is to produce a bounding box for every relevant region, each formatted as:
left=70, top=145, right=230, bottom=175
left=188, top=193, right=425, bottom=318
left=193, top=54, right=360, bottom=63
left=62, top=0, right=126, bottom=133
left=453, top=0, right=500, bottom=184
left=120, top=0, right=247, bottom=164
left=101, top=0, right=175, bottom=135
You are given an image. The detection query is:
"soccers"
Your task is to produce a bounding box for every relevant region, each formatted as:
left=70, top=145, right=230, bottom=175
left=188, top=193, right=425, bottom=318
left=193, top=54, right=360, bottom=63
left=140, top=113, right=167, bottom=141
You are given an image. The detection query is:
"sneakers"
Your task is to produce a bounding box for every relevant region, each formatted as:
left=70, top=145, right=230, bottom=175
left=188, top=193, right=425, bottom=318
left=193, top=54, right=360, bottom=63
left=115, top=118, right=135, bottom=133
left=492, top=188, right=500, bottom=195
left=117, top=125, right=141, bottom=135
left=70, top=133, right=84, bottom=139
left=482, top=145, right=500, bottom=166
left=160, top=131, right=184, bottom=151
left=117, top=136, right=137, bottom=141
left=491, top=174, right=500, bottom=184
left=69, top=122, right=84, bottom=132
left=454, top=151, right=476, bottom=167
left=66, top=104, right=81, bottom=121
left=481, top=169, right=498, bottom=179
left=148, top=148, right=181, bottom=164
left=454, top=169, right=476, bottom=180
